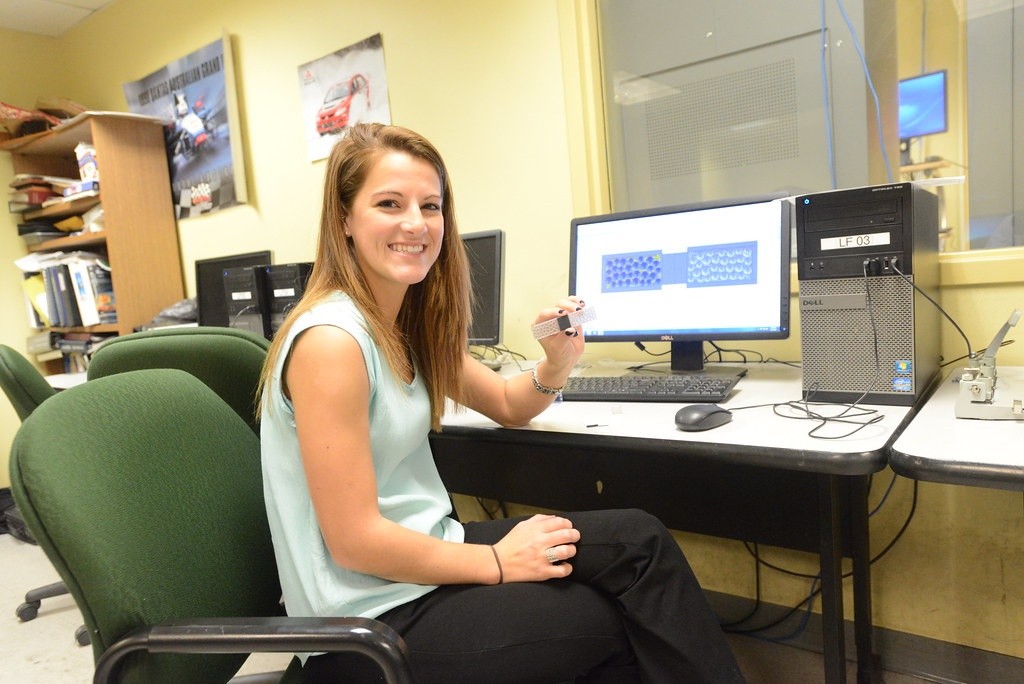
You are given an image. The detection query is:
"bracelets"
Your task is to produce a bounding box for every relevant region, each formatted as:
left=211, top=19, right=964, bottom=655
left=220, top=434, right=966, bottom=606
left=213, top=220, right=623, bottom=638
left=532, top=360, right=565, bottom=395
left=491, top=545, right=503, bottom=585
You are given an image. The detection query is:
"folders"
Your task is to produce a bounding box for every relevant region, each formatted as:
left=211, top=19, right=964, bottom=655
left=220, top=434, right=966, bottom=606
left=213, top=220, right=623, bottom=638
left=40, top=263, right=80, bottom=328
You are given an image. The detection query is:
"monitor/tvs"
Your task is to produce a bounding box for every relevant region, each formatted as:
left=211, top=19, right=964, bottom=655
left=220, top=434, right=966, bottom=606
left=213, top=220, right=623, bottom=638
left=194, top=250, right=272, bottom=328
left=459, top=229, right=505, bottom=347
left=897, top=68, right=948, bottom=141
left=568, top=191, right=792, bottom=377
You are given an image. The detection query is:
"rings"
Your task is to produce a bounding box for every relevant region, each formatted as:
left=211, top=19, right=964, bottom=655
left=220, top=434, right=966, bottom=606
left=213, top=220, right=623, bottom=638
left=547, top=547, right=558, bottom=562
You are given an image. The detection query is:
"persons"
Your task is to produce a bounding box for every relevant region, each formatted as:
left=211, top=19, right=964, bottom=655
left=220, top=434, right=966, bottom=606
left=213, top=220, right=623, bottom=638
left=255, top=123, right=747, bottom=684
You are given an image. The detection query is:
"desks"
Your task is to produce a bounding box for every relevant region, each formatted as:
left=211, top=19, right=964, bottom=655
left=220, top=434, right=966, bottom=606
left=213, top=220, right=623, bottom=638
left=433, top=359, right=1024, bottom=684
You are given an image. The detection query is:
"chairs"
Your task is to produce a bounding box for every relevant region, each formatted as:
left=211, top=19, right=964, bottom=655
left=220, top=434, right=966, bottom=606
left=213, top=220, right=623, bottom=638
left=0, top=328, right=418, bottom=683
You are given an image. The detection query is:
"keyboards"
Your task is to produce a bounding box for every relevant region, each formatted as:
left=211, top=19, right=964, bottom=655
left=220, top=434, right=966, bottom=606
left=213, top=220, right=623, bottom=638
left=221, top=262, right=315, bottom=343
left=554, top=376, right=740, bottom=403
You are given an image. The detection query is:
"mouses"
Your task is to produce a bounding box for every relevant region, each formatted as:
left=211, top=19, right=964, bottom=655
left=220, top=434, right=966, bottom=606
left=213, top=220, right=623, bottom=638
left=674, top=404, right=733, bottom=432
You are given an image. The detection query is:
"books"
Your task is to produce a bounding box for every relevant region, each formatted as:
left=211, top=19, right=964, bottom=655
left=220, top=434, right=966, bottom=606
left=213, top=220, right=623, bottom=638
left=36, top=95, right=87, bottom=117
left=9, top=174, right=119, bottom=374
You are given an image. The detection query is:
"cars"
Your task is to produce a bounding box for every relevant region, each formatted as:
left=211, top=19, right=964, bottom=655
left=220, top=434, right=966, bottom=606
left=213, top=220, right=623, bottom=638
left=314, top=73, right=371, bottom=138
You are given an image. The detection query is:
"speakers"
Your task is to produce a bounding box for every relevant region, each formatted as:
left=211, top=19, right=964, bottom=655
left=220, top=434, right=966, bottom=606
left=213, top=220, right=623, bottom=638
left=795, top=181, right=944, bottom=408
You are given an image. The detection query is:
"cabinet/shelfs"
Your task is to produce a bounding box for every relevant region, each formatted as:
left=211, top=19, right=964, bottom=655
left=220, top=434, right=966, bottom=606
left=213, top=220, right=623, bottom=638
left=9, top=110, right=187, bottom=388
